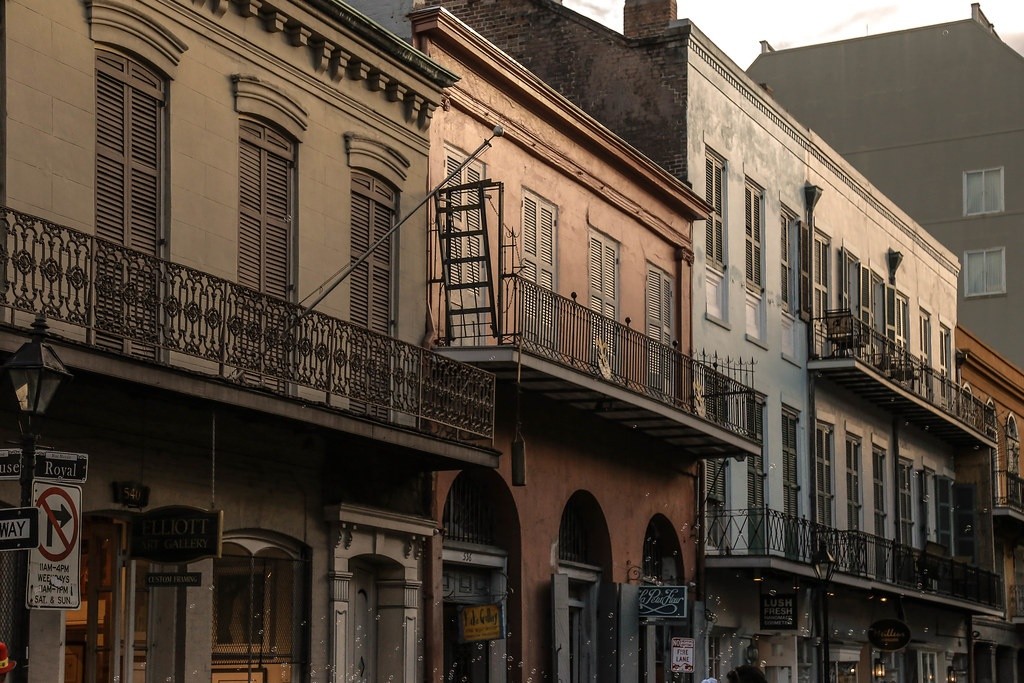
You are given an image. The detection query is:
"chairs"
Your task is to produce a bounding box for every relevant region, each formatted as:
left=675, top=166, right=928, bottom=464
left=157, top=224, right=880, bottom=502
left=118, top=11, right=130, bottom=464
left=826, top=308, right=914, bottom=390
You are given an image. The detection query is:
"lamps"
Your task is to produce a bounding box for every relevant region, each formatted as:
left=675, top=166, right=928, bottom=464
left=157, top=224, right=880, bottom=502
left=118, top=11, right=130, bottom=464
left=873, top=658, right=885, bottom=679
left=738, top=634, right=760, bottom=662
left=948, top=666, right=958, bottom=683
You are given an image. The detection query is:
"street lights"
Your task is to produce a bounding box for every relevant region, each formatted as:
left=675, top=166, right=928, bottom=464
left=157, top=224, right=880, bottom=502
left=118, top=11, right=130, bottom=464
left=809, top=544, right=839, bottom=683
left=9, top=312, right=75, bottom=683
